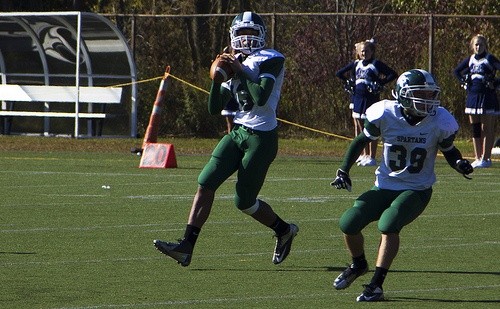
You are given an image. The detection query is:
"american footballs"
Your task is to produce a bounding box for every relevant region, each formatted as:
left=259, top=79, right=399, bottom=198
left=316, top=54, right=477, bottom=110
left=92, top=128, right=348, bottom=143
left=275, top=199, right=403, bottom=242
left=209, top=57, right=233, bottom=82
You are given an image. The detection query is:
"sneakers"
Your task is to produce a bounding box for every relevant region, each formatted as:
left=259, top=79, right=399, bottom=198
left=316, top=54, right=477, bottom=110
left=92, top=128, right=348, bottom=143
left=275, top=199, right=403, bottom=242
left=153, top=238, right=192, bottom=267
left=356, top=284, right=385, bottom=302
left=271, top=223, right=299, bottom=265
left=333, top=260, right=368, bottom=290
left=470, top=157, right=491, bottom=168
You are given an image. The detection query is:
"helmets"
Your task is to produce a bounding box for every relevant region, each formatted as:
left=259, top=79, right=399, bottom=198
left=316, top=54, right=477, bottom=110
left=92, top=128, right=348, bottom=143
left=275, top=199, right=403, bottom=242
left=229, top=11, right=265, bottom=53
left=394, top=68, right=442, bottom=116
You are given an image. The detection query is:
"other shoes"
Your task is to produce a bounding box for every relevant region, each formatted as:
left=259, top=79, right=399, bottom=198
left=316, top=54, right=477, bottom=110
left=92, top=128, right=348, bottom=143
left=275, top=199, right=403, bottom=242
left=363, top=157, right=377, bottom=167
left=355, top=155, right=364, bottom=162
left=357, top=154, right=368, bottom=166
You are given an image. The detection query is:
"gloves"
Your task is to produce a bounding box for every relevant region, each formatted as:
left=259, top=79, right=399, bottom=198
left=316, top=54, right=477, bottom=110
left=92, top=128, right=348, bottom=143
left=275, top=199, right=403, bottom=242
left=456, top=159, right=473, bottom=175
left=330, top=168, right=352, bottom=192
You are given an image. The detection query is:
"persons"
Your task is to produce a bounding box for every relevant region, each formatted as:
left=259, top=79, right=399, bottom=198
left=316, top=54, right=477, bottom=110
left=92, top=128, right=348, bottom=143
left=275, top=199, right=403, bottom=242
left=329, top=68, right=472, bottom=302
left=153, top=12, right=298, bottom=266
left=454, top=35, right=500, bottom=168
left=336, top=40, right=399, bottom=166
